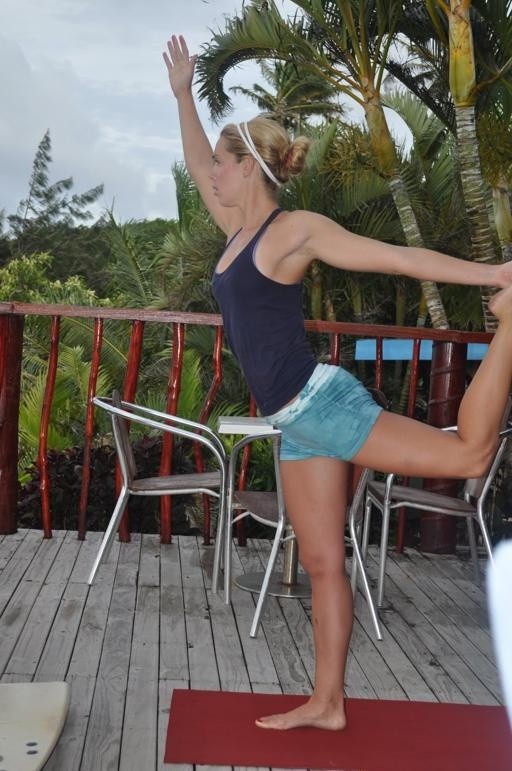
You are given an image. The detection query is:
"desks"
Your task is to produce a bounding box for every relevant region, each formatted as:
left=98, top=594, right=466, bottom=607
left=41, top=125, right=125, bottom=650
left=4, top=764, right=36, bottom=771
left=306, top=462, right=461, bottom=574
left=216, top=413, right=316, bottom=599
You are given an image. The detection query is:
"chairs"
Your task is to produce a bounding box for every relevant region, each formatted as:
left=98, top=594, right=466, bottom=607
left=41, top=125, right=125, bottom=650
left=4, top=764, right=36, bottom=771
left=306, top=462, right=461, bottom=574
left=87, top=394, right=228, bottom=593
left=351, top=425, right=507, bottom=607
left=227, top=435, right=384, bottom=642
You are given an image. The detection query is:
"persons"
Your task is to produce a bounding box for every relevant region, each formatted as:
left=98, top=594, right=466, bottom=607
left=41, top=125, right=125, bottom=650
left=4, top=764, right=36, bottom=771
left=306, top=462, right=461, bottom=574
left=164, top=36, right=512, bottom=732
